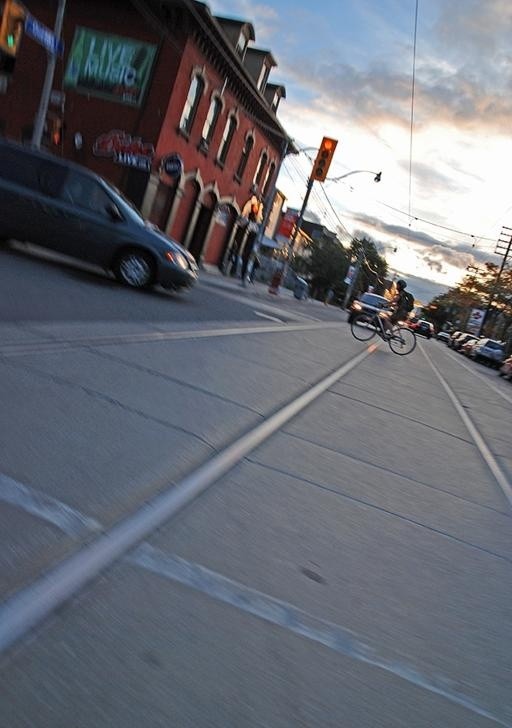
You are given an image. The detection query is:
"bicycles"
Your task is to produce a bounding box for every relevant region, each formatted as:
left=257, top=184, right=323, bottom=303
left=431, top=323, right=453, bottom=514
left=350, top=303, right=417, bottom=354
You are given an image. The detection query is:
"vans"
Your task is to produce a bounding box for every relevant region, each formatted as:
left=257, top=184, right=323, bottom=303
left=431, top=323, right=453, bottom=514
left=0, top=140, right=200, bottom=295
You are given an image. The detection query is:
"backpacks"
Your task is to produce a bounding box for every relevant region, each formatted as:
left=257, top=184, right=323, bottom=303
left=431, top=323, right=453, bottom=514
left=400, top=291, right=415, bottom=312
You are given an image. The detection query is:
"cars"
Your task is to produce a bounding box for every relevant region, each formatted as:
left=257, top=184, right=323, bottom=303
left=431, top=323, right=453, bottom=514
left=409, top=317, right=512, bottom=380
left=348, top=292, right=393, bottom=328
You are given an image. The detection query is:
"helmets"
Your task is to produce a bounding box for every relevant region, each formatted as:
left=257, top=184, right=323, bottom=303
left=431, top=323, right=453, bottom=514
left=397, top=279, right=407, bottom=289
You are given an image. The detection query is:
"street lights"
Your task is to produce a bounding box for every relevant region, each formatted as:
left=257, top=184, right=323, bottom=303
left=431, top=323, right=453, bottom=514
left=341, top=245, right=397, bottom=309
left=268, top=170, right=384, bottom=295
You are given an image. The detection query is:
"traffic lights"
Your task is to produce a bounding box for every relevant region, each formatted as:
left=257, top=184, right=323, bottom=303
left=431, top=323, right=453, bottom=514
left=1, top=1, right=30, bottom=58
left=312, top=137, right=338, bottom=183
left=43, top=112, right=64, bottom=146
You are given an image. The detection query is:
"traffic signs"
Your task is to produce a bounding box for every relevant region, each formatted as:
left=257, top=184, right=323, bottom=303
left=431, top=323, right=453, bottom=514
left=24, top=12, right=66, bottom=58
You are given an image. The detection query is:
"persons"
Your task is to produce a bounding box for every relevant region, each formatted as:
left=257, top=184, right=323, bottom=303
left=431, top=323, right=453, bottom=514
left=249, top=256, right=261, bottom=285
left=239, top=253, right=250, bottom=281
left=315, top=285, right=322, bottom=300
left=323, top=287, right=336, bottom=306
left=376, top=279, right=410, bottom=340
left=309, top=279, right=314, bottom=298
